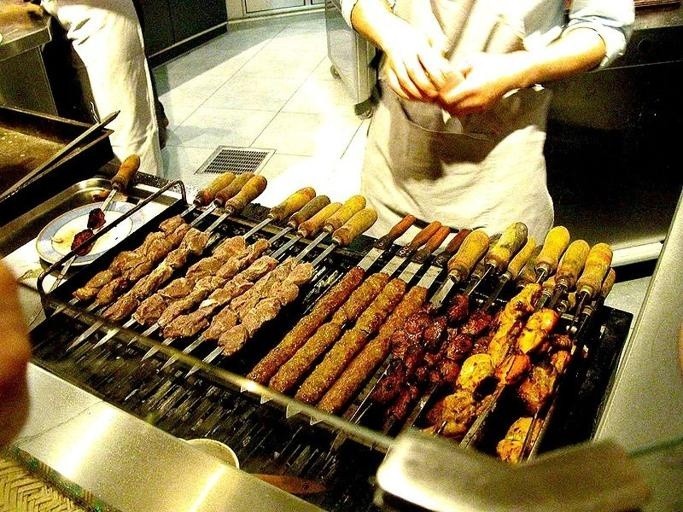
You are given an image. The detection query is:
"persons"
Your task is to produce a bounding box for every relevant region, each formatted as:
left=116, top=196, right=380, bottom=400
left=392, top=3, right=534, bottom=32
left=335, top=0, right=636, bottom=265
left=46, top=0, right=166, bottom=182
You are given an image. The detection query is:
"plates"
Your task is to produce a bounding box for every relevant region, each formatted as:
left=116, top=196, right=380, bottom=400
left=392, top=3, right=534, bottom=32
left=33, top=200, right=144, bottom=267
